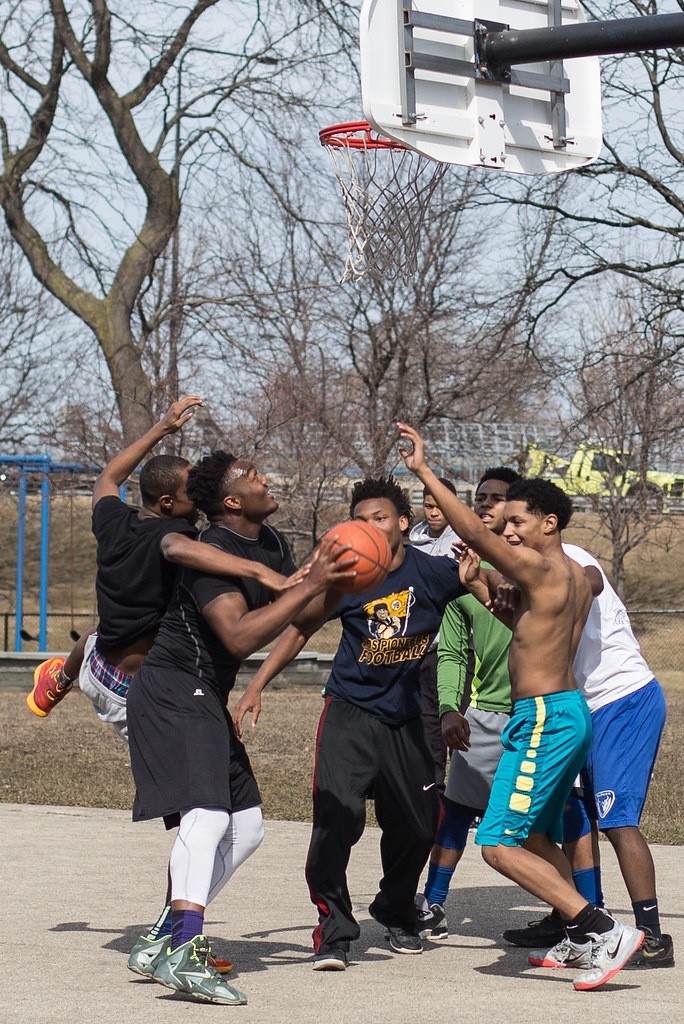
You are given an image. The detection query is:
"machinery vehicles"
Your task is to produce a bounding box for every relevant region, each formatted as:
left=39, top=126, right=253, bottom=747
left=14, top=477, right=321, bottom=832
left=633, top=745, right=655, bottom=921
left=518, top=441, right=684, bottom=514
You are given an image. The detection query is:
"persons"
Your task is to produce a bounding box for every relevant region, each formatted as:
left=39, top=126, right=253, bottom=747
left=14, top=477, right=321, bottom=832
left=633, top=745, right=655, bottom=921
left=233, top=478, right=519, bottom=971
left=27, top=397, right=314, bottom=972
left=124, top=450, right=359, bottom=1005
left=394, top=422, right=675, bottom=990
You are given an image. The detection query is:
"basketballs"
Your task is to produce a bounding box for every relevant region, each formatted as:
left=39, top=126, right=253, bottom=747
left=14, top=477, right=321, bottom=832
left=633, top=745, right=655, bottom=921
left=320, top=519, right=393, bottom=595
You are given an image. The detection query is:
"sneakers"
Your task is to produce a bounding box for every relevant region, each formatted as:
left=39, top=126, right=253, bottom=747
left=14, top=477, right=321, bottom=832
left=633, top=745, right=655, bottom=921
left=27, top=657, right=73, bottom=717
left=368, top=901, right=424, bottom=953
left=383, top=892, right=448, bottom=940
left=312, top=948, right=349, bottom=971
left=528, top=925, right=591, bottom=969
left=620, top=925, right=675, bottom=969
left=573, top=912, right=645, bottom=989
left=126, top=933, right=232, bottom=977
left=503, top=914, right=566, bottom=947
left=153, top=934, right=247, bottom=1005
